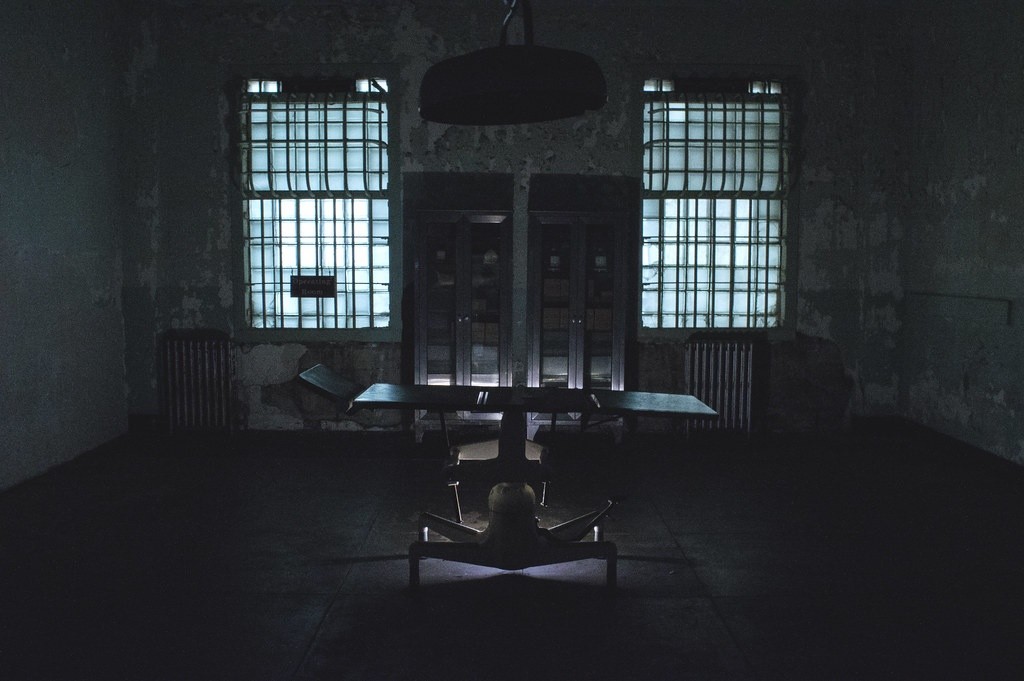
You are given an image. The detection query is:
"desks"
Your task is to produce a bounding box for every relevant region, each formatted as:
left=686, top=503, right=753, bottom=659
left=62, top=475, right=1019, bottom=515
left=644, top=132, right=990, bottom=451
left=350, top=382, right=717, bottom=588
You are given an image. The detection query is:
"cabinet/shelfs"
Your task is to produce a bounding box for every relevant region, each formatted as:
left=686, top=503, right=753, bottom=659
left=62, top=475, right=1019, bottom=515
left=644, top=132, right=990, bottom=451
left=401, top=172, right=638, bottom=443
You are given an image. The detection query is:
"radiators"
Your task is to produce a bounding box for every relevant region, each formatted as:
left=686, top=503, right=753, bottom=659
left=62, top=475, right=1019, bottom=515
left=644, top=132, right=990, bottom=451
left=158, top=326, right=238, bottom=440
left=684, top=331, right=760, bottom=442
